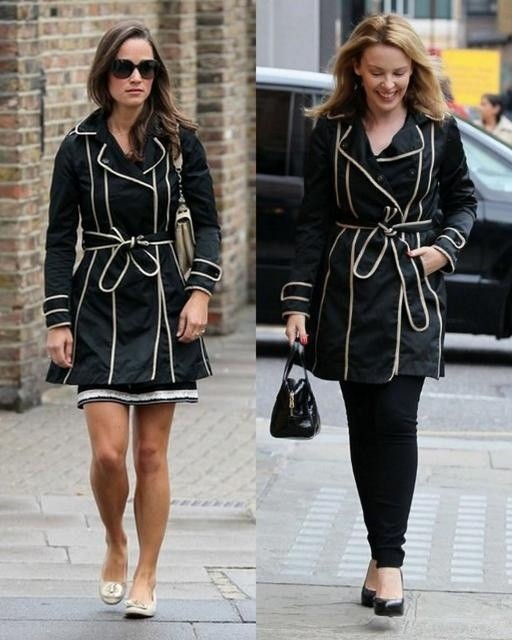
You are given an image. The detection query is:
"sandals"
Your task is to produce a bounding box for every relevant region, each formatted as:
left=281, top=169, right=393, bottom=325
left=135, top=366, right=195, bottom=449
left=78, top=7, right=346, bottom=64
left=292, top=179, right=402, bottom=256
left=124, top=589, right=157, bottom=617
left=100, top=541, right=130, bottom=605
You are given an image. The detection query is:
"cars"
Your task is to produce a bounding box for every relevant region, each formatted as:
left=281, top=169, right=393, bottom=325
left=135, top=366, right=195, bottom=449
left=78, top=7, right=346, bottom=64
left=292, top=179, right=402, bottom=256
left=254, top=65, right=511, bottom=341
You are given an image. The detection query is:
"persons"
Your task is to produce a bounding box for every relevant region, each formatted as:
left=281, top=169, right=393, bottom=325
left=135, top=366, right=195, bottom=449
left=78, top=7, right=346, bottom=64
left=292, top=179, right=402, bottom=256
left=278, top=12, right=478, bottom=618
left=42, top=18, right=223, bottom=618
left=437, top=75, right=468, bottom=119
left=471, top=95, right=511, bottom=148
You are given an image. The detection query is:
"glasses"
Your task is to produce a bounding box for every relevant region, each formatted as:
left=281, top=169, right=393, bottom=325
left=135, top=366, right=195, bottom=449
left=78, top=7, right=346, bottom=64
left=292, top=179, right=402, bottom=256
left=108, top=60, right=159, bottom=79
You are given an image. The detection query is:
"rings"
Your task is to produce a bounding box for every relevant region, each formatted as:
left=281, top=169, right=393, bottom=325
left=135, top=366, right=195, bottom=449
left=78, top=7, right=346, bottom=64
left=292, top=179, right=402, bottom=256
left=196, top=331, right=201, bottom=337
left=200, top=329, right=206, bottom=334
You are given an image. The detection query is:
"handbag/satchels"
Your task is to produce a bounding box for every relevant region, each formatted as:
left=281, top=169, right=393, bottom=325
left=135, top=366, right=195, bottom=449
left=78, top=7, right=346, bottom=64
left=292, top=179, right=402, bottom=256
left=175, top=204, right=196, bottom=281
left=271, top=378, right=320, bottom=438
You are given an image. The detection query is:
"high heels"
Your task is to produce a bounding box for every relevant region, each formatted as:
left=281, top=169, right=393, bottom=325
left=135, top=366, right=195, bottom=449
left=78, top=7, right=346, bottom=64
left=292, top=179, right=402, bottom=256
left=361, top=569, right=404, bottom=617
